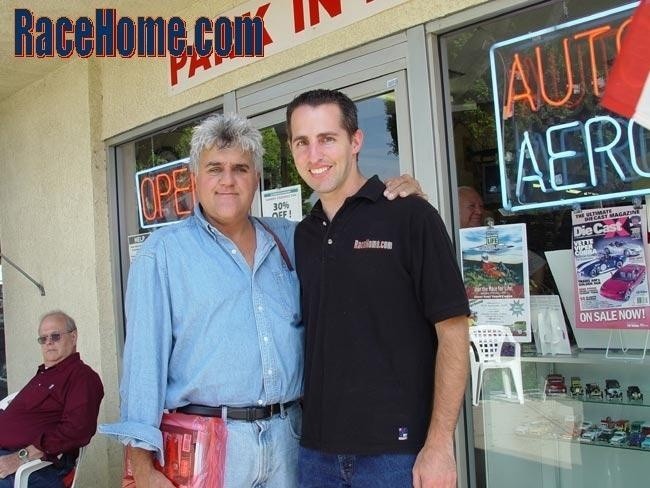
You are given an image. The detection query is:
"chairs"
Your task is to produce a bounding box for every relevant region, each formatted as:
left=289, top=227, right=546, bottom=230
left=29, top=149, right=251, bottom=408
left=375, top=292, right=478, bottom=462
left=0, top=392, right=83, bottom=488
left=467, top=325, right=525, bottom=407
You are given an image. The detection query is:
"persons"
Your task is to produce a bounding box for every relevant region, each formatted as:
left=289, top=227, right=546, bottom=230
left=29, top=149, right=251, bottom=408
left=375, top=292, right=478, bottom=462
left=0, top=309, right=108, bottom=488
left=98, top=112, right=428, bottom=488
left=285, top=86, right=473, bottom=487
left=459, top=184, right=545, bottom=297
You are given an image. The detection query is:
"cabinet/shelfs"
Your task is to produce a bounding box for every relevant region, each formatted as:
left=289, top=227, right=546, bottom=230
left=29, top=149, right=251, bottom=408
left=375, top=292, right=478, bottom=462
left=482, top=351, right=650, bottom=488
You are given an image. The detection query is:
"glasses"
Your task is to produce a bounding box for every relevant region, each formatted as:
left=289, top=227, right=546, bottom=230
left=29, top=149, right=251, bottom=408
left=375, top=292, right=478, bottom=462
left=37, top=329, right=73, bottom=346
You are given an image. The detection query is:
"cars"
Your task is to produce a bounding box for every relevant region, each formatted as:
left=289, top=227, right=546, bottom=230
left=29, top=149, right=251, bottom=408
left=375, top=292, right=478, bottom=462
left=577, top=240, right=647, bottom=301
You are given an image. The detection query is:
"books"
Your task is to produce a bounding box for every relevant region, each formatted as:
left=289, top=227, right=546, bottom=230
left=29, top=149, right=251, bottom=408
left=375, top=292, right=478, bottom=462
left=121, top=415, right=224, bottom=488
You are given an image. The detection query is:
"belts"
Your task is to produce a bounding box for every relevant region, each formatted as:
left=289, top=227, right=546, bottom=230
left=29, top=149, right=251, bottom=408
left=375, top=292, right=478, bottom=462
left=172, top=401, right=299, bottom=422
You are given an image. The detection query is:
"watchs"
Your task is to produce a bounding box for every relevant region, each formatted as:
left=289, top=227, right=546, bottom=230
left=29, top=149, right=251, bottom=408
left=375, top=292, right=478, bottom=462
left=18, top=448, right=31, bottom=465
